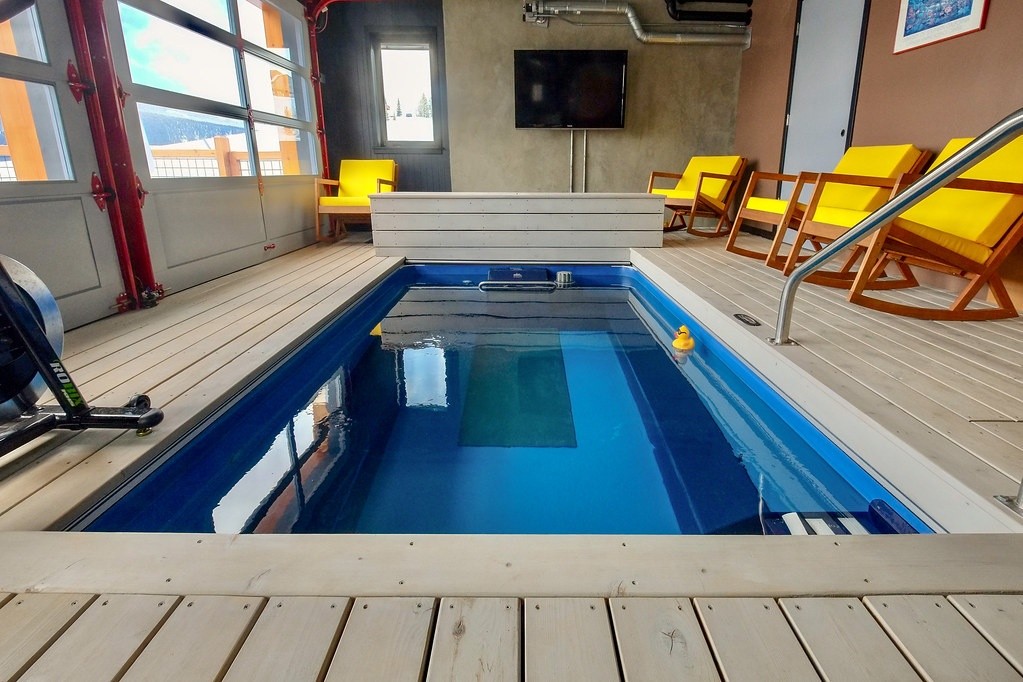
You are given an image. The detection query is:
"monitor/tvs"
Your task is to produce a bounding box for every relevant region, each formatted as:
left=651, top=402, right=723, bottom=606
left=514, top=50, right=629, bottom=130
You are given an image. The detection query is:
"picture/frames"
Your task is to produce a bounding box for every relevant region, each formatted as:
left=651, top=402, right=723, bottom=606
left=888, top=0, right=989, bottom=55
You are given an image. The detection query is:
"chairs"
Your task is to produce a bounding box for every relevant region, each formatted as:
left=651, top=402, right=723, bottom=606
left=783, top=138, right=1022, bottom=323
left=726, top=144, right=932, bottom=279
left=648, top=156, right=747, bottom=239
left=315, top=159, right=399, bottom=244
